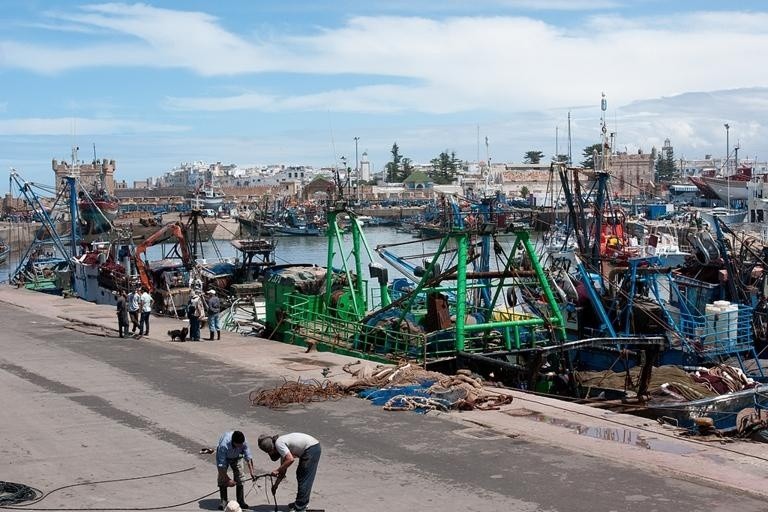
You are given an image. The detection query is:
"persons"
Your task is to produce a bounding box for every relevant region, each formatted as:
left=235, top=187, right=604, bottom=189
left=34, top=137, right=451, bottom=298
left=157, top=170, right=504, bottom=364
left=216, top=430, right=257, bottom=510
left=258, top=432, right=321, bottom=510
left=116, top=289, right=130, bottom=338
left=187, top=290, right=205, bottom=342
left=206, top=290, right=221, bottom=340
left=127, top=287, right=141, bottom=335
left=138, top=286, right=155, bottom=335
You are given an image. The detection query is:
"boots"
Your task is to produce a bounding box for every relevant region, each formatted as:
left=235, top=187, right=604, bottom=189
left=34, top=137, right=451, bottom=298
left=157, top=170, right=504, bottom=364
left=209, top=332, right=214, bottom=341
left=236, top=485, right=248, bottom=508
left=217, top=330, right=220, bottom=340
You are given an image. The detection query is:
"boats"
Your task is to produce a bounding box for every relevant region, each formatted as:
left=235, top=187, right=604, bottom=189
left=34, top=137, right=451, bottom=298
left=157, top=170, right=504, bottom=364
left=0, top=91, right=768, bottom=443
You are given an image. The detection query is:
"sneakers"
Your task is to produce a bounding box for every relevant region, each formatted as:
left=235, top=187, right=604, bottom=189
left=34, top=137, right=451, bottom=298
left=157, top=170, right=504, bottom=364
left=288, top=502, right=306, bottom=512
left=218, top=487, right=227, bottom=511
left=119, top=325, right=149, bottom=338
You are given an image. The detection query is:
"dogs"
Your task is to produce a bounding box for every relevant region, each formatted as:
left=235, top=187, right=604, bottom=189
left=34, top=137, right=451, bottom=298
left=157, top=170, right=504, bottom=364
left=167, top=327, right=189, bottom=342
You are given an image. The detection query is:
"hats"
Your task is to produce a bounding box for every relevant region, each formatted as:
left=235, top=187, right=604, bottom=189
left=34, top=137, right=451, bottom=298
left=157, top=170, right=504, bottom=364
left=195, top=290, right=202, bottom=293
left=223, top=501, right=242, bottom=512
left=207, top=289, right=216, bottom=295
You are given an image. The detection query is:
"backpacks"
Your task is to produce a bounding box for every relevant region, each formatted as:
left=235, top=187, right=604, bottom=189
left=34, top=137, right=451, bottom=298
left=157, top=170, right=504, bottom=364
left=186, top=305, right=195, bottom=315
